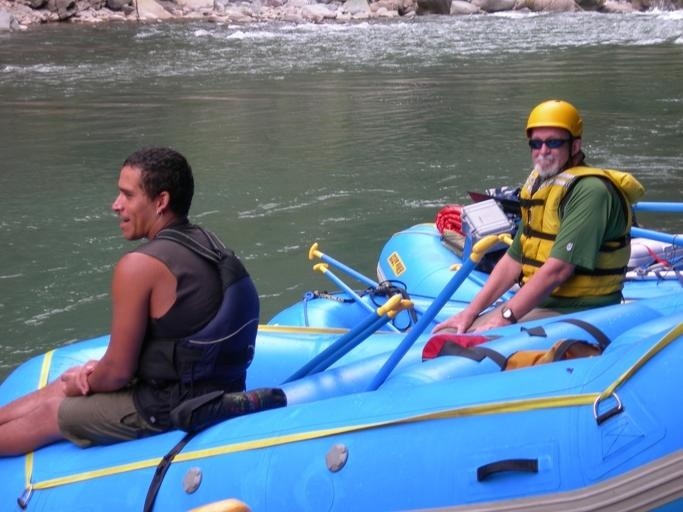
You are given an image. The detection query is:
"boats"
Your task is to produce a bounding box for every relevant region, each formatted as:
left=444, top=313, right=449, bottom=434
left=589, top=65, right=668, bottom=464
left=266, top=290, right=511, bottom=335
left=373, top=205, right=683, bottom=302
left=0, top=325, right=680, bottom=512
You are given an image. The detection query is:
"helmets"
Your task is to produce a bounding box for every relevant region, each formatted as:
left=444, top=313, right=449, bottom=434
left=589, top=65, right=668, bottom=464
left=525, top=99, right=583, bottom=139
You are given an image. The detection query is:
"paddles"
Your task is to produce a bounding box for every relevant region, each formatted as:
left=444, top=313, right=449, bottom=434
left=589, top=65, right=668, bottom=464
left=468, top=192, right=683, bottom=246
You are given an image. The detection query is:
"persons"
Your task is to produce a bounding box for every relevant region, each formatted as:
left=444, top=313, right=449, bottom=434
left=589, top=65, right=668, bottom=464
left=431, top=99, right=645, bottom=338
left=0, top=147, right=258, bottom=460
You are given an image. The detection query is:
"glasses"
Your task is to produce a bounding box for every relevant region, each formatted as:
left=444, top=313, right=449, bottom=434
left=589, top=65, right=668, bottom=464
left=529, top=138, right=578, bottom=150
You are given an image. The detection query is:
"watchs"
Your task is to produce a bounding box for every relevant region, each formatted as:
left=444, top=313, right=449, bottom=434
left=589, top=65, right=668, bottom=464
left=500, top=306, right=518, bottom=324
